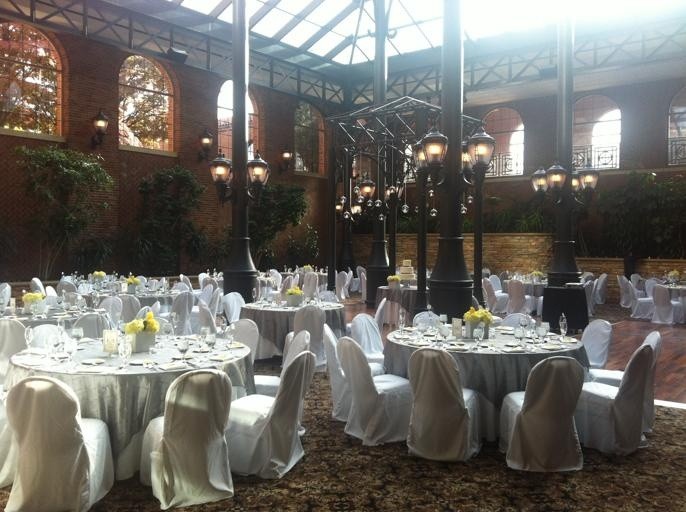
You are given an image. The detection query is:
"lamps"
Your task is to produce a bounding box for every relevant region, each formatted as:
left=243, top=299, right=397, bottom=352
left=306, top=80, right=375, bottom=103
left=197, top=127, right=215, bottom=163
left=278, top=146, right=293, bottom=176
left=87, top=110, right=112, bottom=148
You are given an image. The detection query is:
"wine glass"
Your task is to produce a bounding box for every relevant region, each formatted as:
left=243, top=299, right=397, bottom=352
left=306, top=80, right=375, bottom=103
left=510, top=270, right=543, bottom=283
left=258, top=264, right=330, bottom=309
left=3, top=270, right=236, bottom=365
left=395, top=307, right=568, bottom=349
